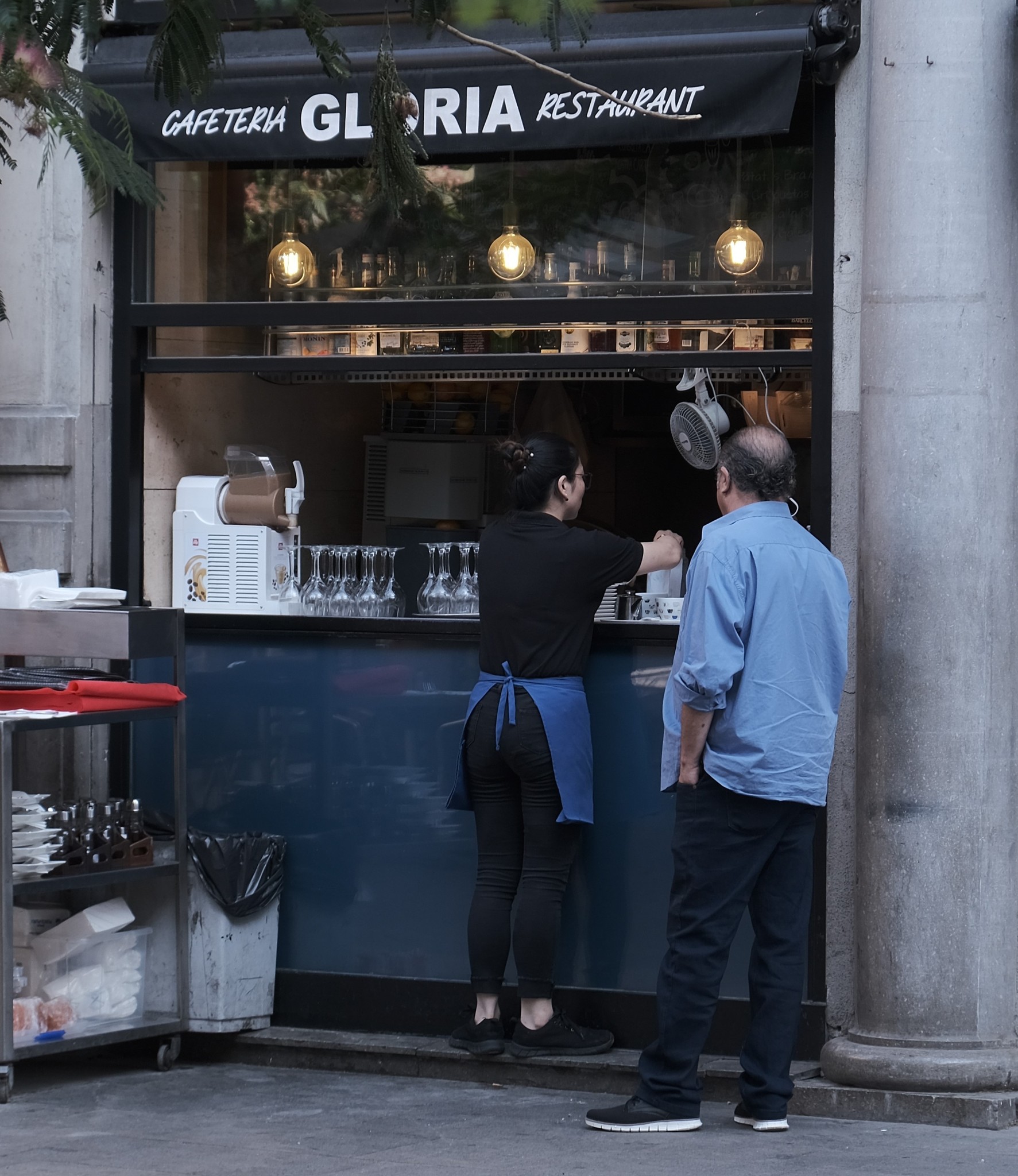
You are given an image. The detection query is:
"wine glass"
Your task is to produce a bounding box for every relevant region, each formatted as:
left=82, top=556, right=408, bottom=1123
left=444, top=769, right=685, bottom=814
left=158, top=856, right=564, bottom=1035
left=417, top=542, right=480, bottom=613
left=277, top=544, right=405, bottom=617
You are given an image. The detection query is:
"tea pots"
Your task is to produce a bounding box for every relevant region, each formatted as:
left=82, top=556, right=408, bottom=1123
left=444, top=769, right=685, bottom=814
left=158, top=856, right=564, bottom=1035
left=615, top=589, right=642, bottom=620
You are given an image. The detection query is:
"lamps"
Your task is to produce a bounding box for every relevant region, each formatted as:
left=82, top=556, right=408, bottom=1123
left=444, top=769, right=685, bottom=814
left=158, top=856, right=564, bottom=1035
left=488, top=158, right=537, bottom=281
left=716, top=138, right=764, bottom=277
left=267, top=166, right=314, bottom=289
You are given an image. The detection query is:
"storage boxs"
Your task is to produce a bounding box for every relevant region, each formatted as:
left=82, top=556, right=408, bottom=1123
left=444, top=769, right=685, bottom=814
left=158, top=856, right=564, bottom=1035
left=12, top=896, right=154, bottom=1031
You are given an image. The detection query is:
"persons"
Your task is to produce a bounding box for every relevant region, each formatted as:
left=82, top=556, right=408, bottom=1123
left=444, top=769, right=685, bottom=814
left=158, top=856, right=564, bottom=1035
left=445, top=435, right=618, bottom=1058
left=583, top=423, right=852, bottom=1134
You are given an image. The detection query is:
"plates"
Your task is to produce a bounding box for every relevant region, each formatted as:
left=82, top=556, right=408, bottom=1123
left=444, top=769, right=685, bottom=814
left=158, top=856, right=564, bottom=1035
left=412, top=612, right=480, bottom=619
left=11, top=789, right=66, bottom=878
left=594, top=588, right=618, bottom=621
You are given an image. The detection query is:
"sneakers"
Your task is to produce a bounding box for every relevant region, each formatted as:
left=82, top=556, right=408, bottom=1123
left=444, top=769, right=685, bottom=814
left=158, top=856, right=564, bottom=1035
left=734, top=1100, right=789, bottom=1131
left=585, top=1095, right=703, bottom=1133
left=509, top=1007, right=615, bottom=1057
left=448, top=998, right=505, bottom=1055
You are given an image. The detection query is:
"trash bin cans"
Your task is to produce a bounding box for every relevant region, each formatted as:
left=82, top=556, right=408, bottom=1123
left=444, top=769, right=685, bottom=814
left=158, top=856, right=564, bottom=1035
left=135, top=832, right=286, bottom=1034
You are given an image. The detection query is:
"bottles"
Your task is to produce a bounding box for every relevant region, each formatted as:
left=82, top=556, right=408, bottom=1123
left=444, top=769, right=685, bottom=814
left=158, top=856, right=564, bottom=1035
left=45, top=795, right=144, bottom=868
left=274, top=239, right=811, bottom=356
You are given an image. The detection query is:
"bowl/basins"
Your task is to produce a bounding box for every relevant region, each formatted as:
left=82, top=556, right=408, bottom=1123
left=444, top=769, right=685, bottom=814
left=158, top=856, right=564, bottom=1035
left=654, top=597, right=684, bottom=622
left=635, top=593, right=667, bottom=618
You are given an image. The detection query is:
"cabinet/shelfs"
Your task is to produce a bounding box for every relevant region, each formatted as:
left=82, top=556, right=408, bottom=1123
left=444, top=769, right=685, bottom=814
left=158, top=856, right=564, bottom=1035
left=0, top=605, right=192, bottom=1108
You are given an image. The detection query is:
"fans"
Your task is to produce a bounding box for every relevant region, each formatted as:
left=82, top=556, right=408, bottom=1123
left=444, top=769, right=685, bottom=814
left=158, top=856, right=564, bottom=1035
left=669, top=366, right=730, bottom=469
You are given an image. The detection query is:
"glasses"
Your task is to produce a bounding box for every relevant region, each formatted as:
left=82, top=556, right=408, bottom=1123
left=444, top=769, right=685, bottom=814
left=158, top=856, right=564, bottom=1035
left=554, top=472, right=592, bottom=495
